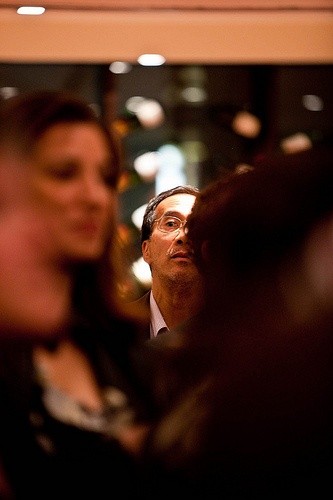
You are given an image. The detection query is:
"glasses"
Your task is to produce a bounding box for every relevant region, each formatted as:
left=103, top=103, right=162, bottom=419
left=153, top=217, right=190, bottom=232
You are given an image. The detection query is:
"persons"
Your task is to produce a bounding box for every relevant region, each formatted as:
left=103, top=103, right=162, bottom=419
left=0, top=88, right=176, bottom=500
left=128, top=186, right=205, bottom=340
left=141, top=143, right=332, bottom=500
